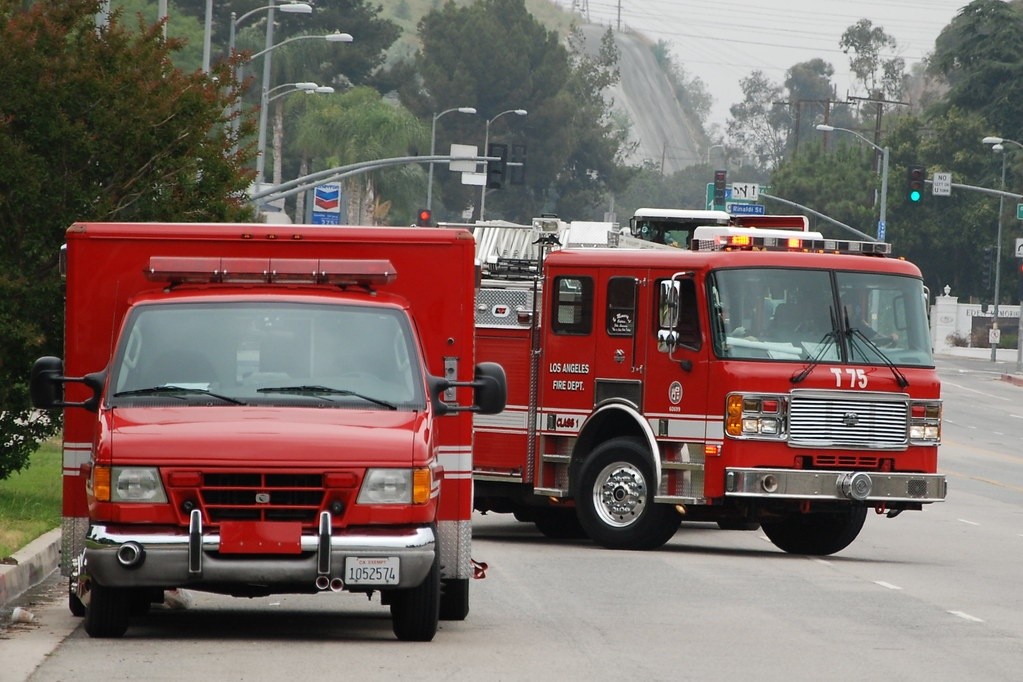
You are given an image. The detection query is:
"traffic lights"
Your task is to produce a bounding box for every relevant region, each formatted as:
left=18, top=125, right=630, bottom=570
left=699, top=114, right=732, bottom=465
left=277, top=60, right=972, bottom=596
left=714, top=170, right=726, bottom=206
left=416, top=209, right=431, bottom=227
left=906, top=165, right=926, bottom=206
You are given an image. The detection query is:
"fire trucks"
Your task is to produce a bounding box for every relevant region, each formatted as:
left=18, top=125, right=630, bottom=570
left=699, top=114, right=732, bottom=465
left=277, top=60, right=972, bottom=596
left=30, top=222, right=506, bottom=644
left=473, top=214, right=947, bottom=556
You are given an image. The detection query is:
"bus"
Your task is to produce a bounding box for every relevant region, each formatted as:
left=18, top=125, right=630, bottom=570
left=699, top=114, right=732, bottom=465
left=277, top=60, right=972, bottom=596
left=618, top=208, right=824, bottom=251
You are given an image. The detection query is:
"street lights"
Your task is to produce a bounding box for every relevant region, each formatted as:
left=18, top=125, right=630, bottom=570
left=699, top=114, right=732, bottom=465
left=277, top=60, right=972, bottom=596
left=217, top=4, right=312, bottom=202
left=816, top=124, right=888, bottom=242
left=427, top=108, right=477, bottom=210
left=227, top=34, right=353, bottom=198
left=480, top=110, right=528, bottom=223
